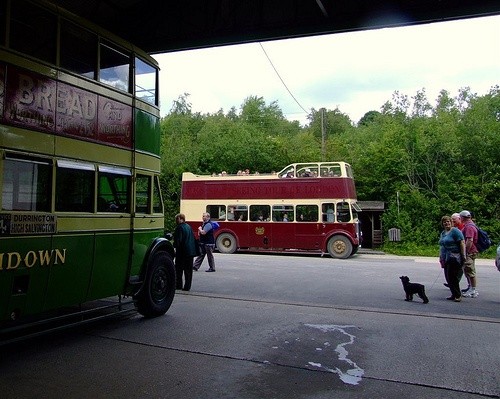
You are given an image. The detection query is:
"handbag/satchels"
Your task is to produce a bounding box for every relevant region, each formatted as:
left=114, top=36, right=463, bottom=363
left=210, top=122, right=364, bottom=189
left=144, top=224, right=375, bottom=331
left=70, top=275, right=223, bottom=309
left=210, top=221, right=220, bottom=231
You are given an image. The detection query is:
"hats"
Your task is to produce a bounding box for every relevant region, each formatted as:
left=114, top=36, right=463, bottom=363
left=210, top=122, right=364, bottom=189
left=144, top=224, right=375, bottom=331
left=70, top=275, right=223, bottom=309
left=460, top=210, right=471, bottom=218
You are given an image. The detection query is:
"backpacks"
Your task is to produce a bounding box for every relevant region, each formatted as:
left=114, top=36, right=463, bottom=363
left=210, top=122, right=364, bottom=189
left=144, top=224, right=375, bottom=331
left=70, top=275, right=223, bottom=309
left=467, top=223, right=492, bottom=253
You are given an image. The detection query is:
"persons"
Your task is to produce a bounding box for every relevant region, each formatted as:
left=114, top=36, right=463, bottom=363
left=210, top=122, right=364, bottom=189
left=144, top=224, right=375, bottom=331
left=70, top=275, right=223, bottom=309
left=192, top=213, right=215, bottom=272
left=264, top=216, right=270, bottom=221
left=298, top=214, right=304, bottom=221
left=257, top=214, right=263, bottom=221
left=285, top=168, right=333, bottom=178
left=173, top=214, right=196, bottom=291
left=272, top=216, right=277, bottom=221
left=211, top=168, right=278, bottom=178
left=460, top=210, right=479, bottom=298
left=443, top=213, right=471, bottom=292
left=237, top=214, right=244, bottom=221
left=283, top=213, right=288, bottom=221
left=219, top=211, right=226, bottom=219
left=440, top=216, right=466, bottom=301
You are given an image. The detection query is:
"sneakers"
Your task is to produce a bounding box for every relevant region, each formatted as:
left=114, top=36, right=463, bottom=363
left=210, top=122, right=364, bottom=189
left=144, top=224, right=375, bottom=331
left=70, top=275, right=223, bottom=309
left=443, top=283, right=450, bottom=287
left=461, top=287, right=470, bottom=291
left=462, top=291, right=477, bottom=298
left=455, top=296, right=461, bottom=301
left=465, top=288, right=480, bottom=296
left=447, top=295, right=454, bottom=300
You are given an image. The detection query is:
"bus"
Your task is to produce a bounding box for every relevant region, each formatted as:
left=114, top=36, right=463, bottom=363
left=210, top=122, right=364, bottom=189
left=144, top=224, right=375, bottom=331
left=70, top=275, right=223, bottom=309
left=0, top=0, right=177, bottom=346
left=179, top=161, right=363, bottom=259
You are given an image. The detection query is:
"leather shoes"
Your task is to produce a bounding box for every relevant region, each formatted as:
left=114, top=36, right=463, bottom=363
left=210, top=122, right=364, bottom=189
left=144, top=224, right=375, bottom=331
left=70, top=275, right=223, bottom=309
left=205, top=268, right=215, bottom=272
left=193, top=266, right=198, bottom=271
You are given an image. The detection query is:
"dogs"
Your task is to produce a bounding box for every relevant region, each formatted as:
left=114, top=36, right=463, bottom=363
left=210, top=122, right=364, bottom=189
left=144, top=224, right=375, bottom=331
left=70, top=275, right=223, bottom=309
left=400, top=276, right=429, bottom=304
